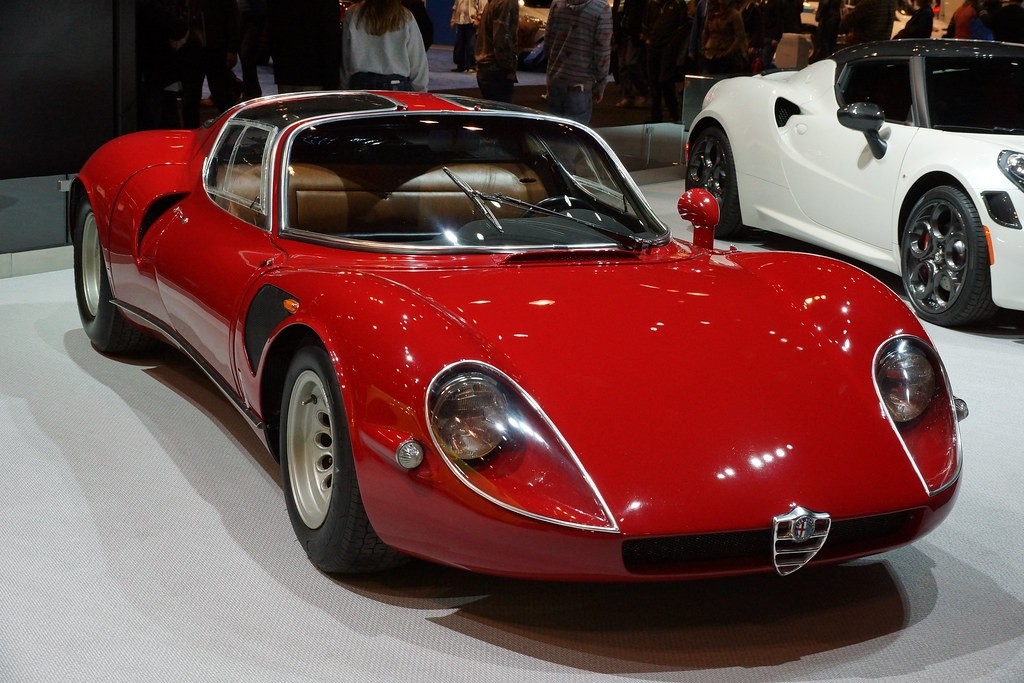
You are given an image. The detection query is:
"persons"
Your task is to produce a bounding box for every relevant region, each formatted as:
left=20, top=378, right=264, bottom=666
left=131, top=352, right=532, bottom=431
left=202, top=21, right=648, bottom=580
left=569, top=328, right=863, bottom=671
left=943, top=0, right=1024, bottom=44
left=542, top=0, right=614, bottom=174
left=815, top=0, right=894, bottom=61
left=450, top=0, right=489, bottom=73
left=476, top=0, right=520, bottom=103
left=134, top=0, right=433, bottom=129
left=611, top=0, right=805, bottom=123
left=342, top=0, right=430, bottom=92
left=892, top=0, right=941, bottom=41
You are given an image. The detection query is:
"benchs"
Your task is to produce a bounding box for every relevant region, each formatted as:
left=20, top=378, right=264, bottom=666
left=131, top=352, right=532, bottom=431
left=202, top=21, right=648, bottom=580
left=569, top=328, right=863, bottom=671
left=227, top=163, right=528, bottom=241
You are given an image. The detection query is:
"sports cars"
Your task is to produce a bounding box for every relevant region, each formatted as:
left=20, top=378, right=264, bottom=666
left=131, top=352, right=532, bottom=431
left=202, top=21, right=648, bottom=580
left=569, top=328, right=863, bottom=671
left=682, top=39, right=1024, bottom=328
left=64, top=88, right=967, bottom=593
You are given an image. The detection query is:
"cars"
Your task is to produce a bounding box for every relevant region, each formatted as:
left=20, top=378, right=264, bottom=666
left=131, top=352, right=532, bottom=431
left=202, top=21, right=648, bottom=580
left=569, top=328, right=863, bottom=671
left=891, top=2, right=948, bottom=42
left=517, top=0, right=553, bottom=45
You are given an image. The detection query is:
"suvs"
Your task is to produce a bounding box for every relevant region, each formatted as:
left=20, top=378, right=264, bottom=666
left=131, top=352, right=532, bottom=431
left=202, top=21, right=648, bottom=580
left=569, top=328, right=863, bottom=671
left=800, top=0, right=820, bottom=28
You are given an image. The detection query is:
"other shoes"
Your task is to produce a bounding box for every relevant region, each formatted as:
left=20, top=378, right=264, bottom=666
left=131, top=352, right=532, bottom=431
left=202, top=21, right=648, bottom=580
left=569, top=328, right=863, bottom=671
left=451, top=67, right=469, bottom=72
left=669, top=112, right=679, bottom=122
